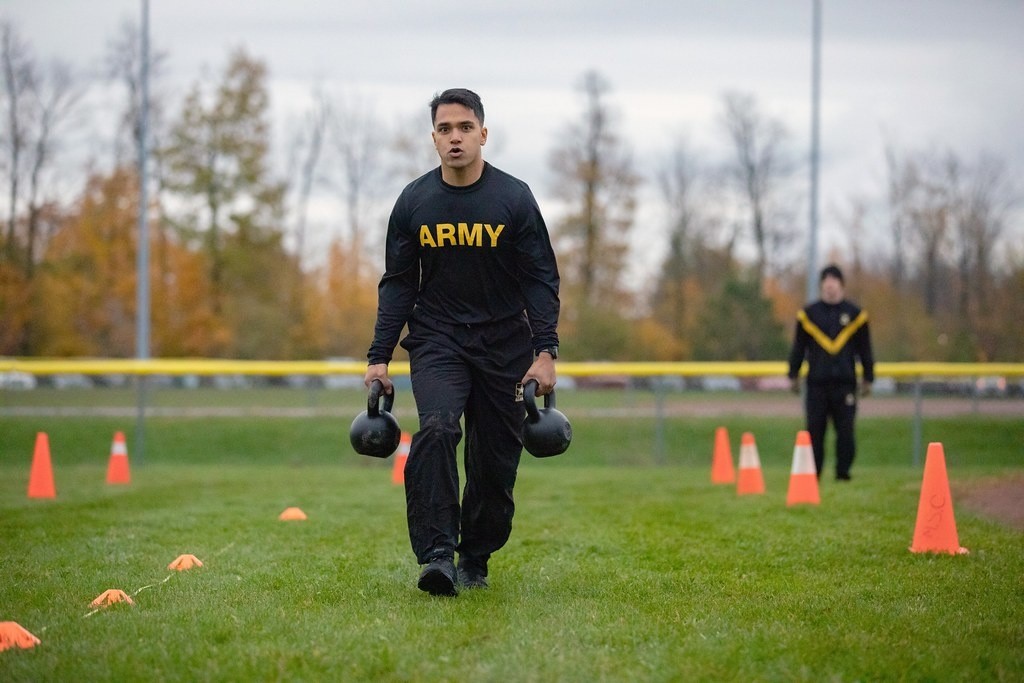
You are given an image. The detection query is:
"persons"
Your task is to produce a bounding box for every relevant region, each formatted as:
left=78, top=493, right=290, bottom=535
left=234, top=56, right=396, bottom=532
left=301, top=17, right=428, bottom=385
left=788, top=263, right=874, bottom=482
left=363, top=88, right=562, bottom=597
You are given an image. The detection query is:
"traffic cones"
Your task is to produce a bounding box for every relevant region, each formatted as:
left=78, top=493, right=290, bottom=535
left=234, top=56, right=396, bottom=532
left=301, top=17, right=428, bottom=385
left=391, top=432, right=413, bottom=485
left=909, top=442, right=969, bottom=555
left=712, top=427, right=736, bottom=484
left=786, top=431, right=823, bottom=505
left=28, top=430, right=54, bottom=496
left=108, top=431, right=131, bottom=483
left=738, top=431, right=764, bottom=496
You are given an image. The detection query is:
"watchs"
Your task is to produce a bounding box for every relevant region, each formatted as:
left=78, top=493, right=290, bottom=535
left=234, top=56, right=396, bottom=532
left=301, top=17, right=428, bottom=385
left=540, top=345, right=558, bottom=360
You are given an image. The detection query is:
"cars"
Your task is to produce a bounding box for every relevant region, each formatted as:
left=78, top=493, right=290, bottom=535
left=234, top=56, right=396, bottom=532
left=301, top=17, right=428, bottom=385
left=1, top=372, right=360, bottom=392
left=973, top=377, right=1007, bottom=397
left=553, top=375, right=897, bottom=394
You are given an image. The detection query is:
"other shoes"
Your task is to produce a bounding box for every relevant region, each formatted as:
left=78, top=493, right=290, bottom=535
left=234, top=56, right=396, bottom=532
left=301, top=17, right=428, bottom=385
left=456, top=555, right=488, bottom=590
left=418, top=556, right=458, bottom=597
